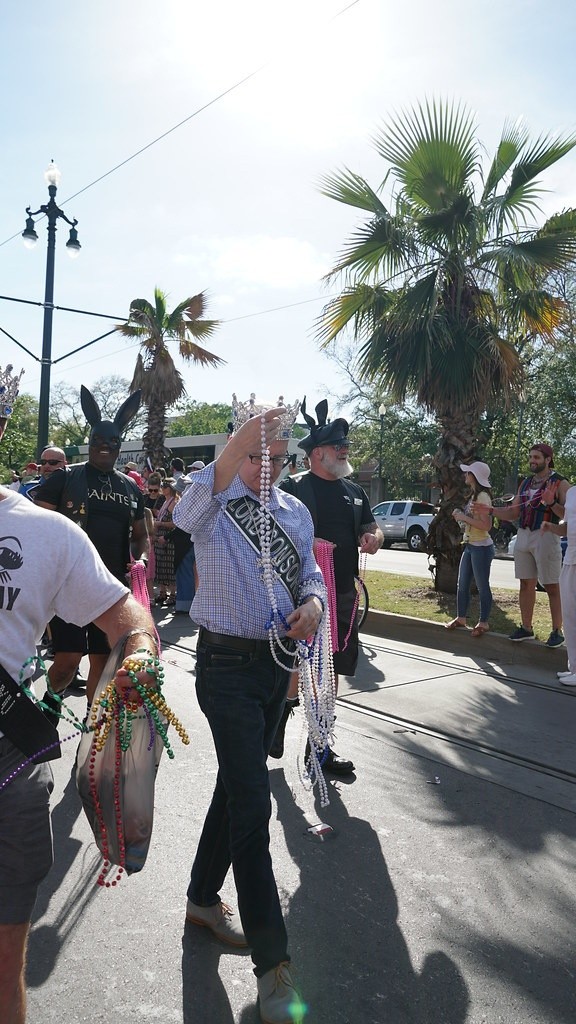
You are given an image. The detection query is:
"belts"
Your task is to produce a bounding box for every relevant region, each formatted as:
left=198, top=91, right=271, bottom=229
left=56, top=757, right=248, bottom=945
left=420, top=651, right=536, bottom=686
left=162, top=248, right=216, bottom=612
left=198, top=623, right=291, bottom=656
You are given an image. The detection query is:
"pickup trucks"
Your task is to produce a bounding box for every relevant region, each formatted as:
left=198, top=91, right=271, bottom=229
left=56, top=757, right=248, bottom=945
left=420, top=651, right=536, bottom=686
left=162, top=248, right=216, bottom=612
left=370, top=500, right=439, bottom=553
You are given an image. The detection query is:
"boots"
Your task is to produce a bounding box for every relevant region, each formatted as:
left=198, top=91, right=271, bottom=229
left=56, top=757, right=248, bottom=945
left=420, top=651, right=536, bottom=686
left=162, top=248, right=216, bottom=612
left=269, top=698, right=300, bottom=760
left=304, top=715, right=356, bottom=775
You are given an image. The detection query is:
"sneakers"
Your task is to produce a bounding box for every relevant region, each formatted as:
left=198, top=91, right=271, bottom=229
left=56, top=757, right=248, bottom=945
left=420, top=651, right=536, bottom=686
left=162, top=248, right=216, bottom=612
left=545, top=630, right=565, bottom=648
left=508, top=625, right=535, bottom=642
left=557, top=671, right=573, bottom=677
left=559, top=674, right=576, bottom=687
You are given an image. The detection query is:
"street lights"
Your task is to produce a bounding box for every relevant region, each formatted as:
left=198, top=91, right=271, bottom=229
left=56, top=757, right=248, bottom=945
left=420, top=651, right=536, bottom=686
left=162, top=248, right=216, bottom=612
left=378, top=402, right=386, bottom=476
left=19, top=158, right=82, bottom=465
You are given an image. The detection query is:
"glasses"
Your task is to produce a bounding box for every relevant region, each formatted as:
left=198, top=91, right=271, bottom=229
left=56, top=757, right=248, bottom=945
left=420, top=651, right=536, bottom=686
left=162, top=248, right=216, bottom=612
left=315, top=443, right=350, bottom=452
left=147, top=489, right=159, bottom=493
left=160, top=484, right=170, bottom=489
left=38, top=459, right=62, bottom=465
left=249, top=450, right=290, bottom=467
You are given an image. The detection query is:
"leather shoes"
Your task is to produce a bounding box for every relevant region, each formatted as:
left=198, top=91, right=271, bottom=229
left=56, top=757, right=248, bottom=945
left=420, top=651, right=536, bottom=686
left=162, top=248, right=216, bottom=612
left=185, top=897, right=249, bottom=948
left=257, top=962, right=302, bottom=1024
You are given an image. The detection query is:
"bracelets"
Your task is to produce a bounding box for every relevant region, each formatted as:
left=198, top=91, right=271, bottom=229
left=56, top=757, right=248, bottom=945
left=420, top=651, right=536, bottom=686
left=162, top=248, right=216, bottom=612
left=548, top=501, right=558, bottom=507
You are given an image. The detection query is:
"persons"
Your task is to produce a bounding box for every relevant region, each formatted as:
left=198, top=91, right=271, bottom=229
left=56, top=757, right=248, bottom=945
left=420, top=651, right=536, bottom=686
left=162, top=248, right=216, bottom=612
left=171, top=391, right=326, bottom=1024
left=122, top=457, right=206, bottom=614
left=8, top=446, right=67, bottom=506
left=34, top=421, right=151, bottom=771
left=469, top=444, right=572, bottom=648
left=445, top=461, right=497, bottom=638
left=540, top=486, right=576, bottom=686
left=277, top=418, right=385, bottom=776
left=0, top=365, right=162, bottom=1024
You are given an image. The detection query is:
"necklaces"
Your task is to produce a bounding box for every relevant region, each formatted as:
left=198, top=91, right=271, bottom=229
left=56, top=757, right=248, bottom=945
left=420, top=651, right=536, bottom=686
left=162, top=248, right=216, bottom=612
left=259, top=409, right=368, bottom=806
left=0, top=648, right=191, bottom=888
left=490, top=470, right=549, bottom=510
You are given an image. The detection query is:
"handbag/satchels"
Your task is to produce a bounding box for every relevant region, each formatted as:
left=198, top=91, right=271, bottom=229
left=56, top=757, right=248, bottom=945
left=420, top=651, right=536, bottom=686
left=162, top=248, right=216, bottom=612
left=73, top=629, right=170, bottom=876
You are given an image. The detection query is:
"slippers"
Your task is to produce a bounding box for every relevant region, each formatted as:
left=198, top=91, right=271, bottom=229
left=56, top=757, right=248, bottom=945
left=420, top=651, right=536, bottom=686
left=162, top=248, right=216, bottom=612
left=445, top=619, right=466, bottom=629
left=471, top=622, right=490, bottom=637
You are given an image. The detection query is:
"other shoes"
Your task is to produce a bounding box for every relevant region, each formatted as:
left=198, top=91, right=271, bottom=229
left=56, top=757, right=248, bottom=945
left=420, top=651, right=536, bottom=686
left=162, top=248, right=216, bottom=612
left=161, top=594, right=177, bottom=606
left=154, top=591, right=167, bottom=602
left=40, top=692, right=62, bottom=729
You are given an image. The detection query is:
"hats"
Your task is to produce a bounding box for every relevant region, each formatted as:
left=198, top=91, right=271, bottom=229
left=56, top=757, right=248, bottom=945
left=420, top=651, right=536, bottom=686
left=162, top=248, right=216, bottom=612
left=23, top=463, right=39, bottom=470
left=187, top=460, right=206, bottom=469
left=460, top=462, right=492, bottom=488
left=297, top=395, right=354, bottom=456
left=122, top=462, right=138, bottom=471
left=530, top=443, right=554, bottom=469
left=170, top=475, right=193, bottom=493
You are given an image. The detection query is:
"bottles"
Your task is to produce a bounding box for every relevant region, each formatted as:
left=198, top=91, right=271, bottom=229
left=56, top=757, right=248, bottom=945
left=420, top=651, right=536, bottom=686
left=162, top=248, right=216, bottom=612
left=453, top=508, right=465, bottom=528
